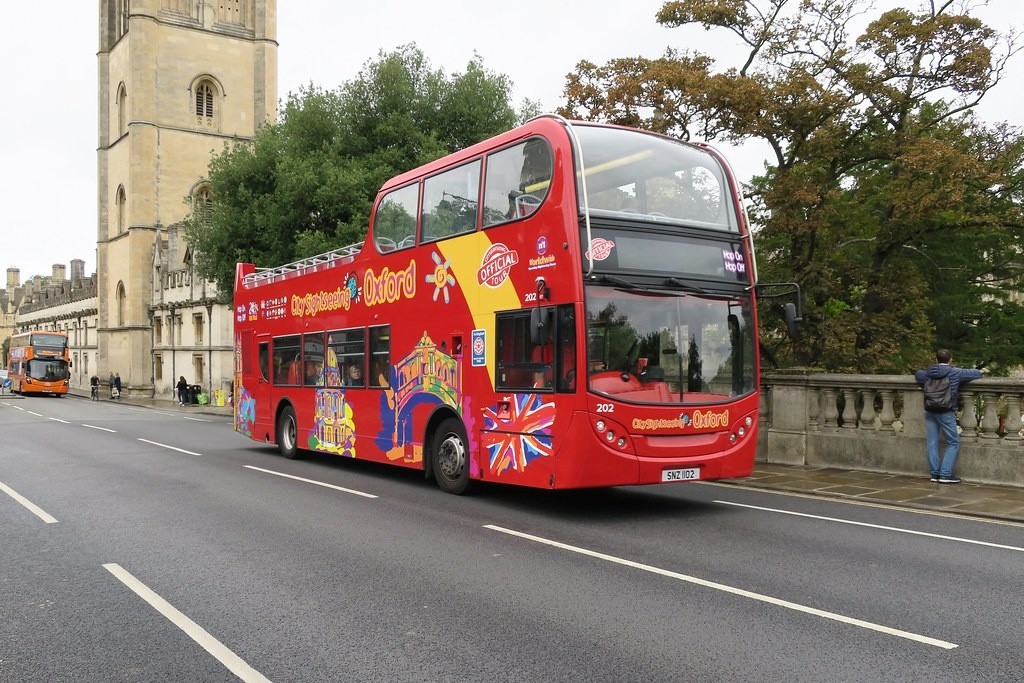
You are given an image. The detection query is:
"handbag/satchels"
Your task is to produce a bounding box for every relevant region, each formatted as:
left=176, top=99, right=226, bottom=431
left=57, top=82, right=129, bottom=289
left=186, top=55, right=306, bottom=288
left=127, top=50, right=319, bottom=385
left=112, top=387, right=119, bottom=397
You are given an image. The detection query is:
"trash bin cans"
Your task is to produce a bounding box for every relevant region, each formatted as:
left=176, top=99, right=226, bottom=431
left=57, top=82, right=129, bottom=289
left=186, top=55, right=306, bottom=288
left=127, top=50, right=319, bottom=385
left=185, top=384, right=191, bottom=403
left=189, top=385, right=201, bottom=404
left=215, top=390, right=224, bottom=406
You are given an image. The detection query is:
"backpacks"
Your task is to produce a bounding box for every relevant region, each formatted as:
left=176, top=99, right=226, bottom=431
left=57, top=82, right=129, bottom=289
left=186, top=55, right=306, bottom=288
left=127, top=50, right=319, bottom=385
left=924, top=376, right=953, bottom=410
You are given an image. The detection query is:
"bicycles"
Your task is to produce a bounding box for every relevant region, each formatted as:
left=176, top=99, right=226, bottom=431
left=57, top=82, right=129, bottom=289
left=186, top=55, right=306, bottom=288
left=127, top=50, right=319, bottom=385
left=90, top=384, right=101, bottom=401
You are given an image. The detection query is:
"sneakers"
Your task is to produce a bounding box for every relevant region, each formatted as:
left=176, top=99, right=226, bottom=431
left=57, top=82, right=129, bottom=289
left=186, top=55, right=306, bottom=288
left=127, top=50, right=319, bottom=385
left=931, top=474, right=960, bottom=482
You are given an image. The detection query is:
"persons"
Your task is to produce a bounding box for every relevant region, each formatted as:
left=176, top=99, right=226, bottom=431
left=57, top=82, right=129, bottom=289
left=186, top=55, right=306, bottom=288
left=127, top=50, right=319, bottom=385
left=176, top=376, right=187, bottom=407
left=230, top=381, right=234, bottom=408
left=69, top=371, right=70, bottom=379
left=531, top=319, right=608, bottom=391
left=915, top=349, right=982, bottom=483
left=91, top=374, right=99, bottom=398
left=109, top=371, right=121, bottom=399
left=263, top=354, right=389, bottom=386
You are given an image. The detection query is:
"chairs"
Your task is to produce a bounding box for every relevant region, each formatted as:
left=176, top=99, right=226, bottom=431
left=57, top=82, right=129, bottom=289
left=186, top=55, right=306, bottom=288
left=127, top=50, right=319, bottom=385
left=244, top=234, right=433, bottom=289
left=515, top=194, right=542, bottom=219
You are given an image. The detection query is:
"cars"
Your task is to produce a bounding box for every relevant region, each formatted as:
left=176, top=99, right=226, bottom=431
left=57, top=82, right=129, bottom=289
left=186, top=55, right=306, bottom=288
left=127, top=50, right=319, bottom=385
left=0, top=370, right=8, bottom=386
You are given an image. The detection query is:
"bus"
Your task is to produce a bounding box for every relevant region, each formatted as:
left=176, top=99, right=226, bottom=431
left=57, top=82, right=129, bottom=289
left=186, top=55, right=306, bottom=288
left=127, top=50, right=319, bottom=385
left=8, top=331, right=72, bottom=397
left=234, top=111, right=803, bottom=490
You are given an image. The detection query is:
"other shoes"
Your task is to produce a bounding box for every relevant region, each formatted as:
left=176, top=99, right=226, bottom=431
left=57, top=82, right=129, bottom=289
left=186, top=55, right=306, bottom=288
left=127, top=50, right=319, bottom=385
left=110, top=397, right=120, bottom=399
left=179, top=402, right=182, bottom=405
left=182, top=404, right=184, bottom=407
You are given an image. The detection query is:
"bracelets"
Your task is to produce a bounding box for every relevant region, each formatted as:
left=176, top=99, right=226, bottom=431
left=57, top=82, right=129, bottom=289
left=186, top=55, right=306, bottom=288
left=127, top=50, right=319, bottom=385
left=593, top=366, right=595, bottom=372
